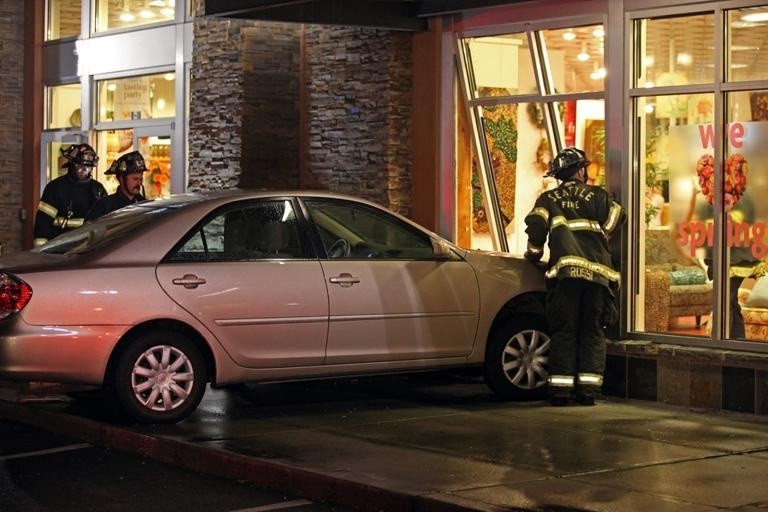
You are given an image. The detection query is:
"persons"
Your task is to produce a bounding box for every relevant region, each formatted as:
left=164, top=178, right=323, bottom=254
left=87, top=152, right=146, bottom=224
left=32, top=143, right=108, bottom=247
left=699, top=194, right=759, bottom=340
left=523, top=148, right=626, bottom=406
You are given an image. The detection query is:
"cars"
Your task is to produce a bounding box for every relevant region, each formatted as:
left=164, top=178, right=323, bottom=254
left=0, top=188, right=551, bottom=426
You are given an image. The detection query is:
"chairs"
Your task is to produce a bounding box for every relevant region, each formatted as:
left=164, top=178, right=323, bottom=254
left=645, top=229, right=768, bottom=342
left=236, top=218, right=295, bottom=258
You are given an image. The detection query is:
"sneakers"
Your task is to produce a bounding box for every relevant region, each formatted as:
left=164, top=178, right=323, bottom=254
left=552, top=390, right=596, bottom=406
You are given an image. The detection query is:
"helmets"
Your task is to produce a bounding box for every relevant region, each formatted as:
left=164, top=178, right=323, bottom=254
left=544, top=147, right=591, bottom=176
left=104, top=151, right=150, bottom=174
left=59, top=144, right=99, bottom=167
